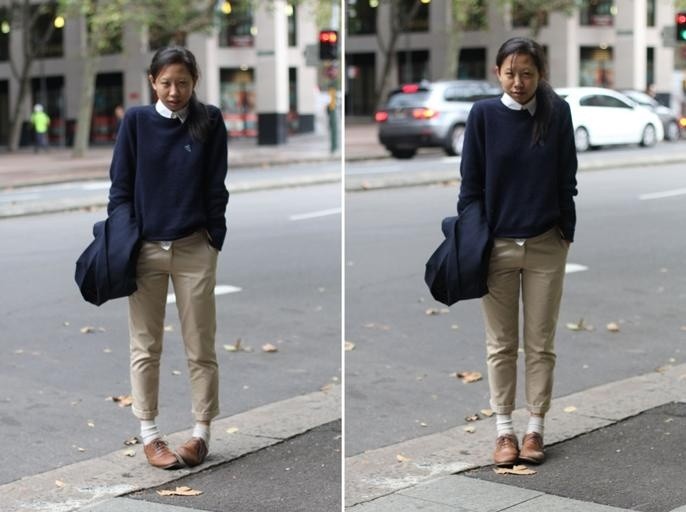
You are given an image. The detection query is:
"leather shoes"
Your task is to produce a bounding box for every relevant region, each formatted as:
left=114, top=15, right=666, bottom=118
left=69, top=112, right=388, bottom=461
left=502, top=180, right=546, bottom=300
left=140, top=436, right=179, bottom=470
left=177, top=436, right=209, bottom=467
left=493, top=432, right=519, bottom=466
left=517, top=431, right=547, bottom=465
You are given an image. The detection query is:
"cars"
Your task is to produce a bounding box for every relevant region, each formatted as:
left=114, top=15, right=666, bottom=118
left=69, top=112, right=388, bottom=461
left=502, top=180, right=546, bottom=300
left=374, top=77, right=686, bottom=156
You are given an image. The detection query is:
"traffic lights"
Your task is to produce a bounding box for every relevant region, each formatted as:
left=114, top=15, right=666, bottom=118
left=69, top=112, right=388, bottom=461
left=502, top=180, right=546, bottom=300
left=674, top=15, right=686, bottom=41
left=321, top=31, right=335, bottom=56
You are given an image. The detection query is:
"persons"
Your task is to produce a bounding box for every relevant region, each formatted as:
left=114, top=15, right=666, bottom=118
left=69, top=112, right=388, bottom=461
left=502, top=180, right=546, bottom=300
left=30, top=104, right=51, bottom=154
left=457, top=36, right=578, bottom=465
left=108, top=46, right=229, bottom=468
